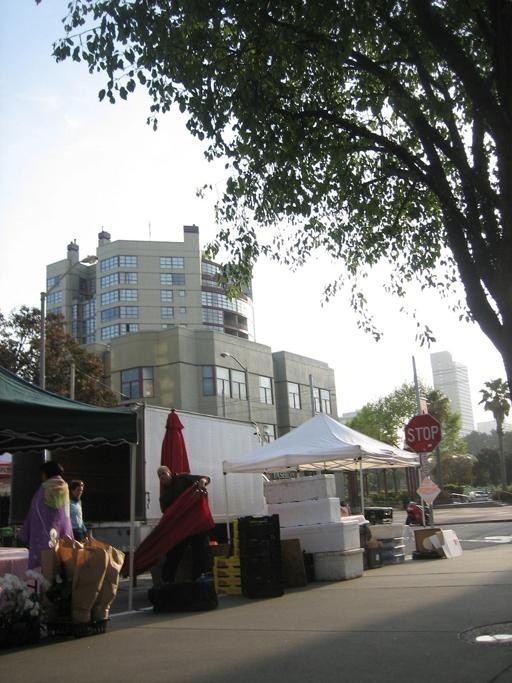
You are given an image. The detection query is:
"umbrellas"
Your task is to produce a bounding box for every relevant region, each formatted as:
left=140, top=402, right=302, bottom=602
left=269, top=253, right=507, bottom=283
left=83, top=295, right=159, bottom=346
left=160, top=407, right=190, bottom=477
left=122, top=480, right=219, bottom=582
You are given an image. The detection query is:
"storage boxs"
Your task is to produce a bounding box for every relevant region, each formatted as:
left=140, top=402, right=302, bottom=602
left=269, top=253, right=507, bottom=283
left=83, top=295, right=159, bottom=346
left=210, top=474, right=446, bottom=598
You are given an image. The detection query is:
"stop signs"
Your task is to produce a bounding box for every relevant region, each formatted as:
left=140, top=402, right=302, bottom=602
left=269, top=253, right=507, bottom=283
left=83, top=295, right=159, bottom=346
left=405, top=412, right=442, bottom=453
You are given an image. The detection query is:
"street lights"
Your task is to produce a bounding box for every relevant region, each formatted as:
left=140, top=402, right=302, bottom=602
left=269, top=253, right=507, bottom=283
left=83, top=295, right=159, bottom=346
left=220, top=350, right=254, bottom=420
left=38, top=254, right=98, bottom=390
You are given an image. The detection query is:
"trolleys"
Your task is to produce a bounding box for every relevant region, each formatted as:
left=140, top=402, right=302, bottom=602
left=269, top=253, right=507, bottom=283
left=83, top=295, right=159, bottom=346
left=35, top=542, right=121, bottom=635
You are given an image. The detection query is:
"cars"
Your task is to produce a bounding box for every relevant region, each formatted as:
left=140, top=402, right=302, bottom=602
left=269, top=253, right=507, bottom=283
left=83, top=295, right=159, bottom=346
left=364, top=506, right=395, bottom=525
left=449, top=490, right=493, bottom=503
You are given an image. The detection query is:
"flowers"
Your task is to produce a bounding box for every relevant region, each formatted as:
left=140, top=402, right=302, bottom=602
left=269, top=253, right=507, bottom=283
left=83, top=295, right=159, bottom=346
left=0, top=570, right=48, bottom=620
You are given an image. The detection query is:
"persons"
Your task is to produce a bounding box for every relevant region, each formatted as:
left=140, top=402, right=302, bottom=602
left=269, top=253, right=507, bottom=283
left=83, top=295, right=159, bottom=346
left=156, top=463, right=211, bottom=580
left=70, top=477, right=90, bottom=544
left=19, top=460, right=75, bottom=570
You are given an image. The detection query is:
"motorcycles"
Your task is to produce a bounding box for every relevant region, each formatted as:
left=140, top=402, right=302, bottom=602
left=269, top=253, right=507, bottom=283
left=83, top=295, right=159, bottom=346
left=407, top=504, right=433, bottom=525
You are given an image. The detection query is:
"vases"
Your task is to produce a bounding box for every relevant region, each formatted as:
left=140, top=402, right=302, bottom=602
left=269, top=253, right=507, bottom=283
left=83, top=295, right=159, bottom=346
left=6, top=621, right=32, bottom=646
